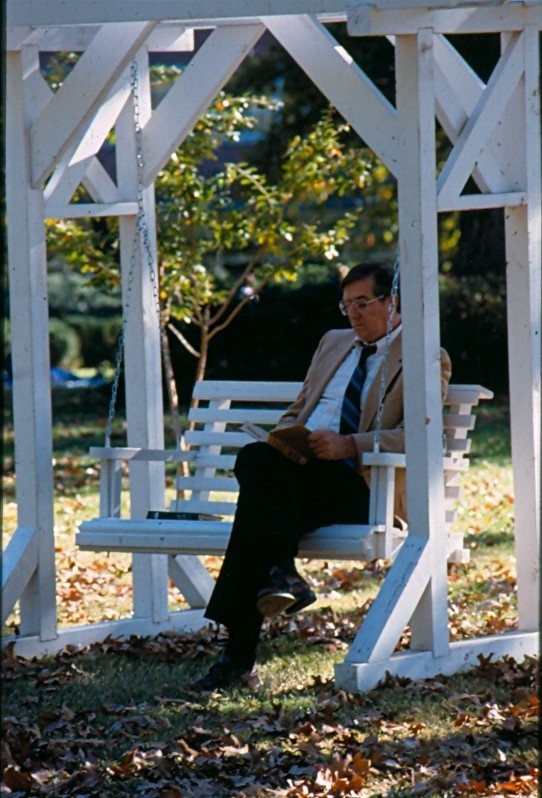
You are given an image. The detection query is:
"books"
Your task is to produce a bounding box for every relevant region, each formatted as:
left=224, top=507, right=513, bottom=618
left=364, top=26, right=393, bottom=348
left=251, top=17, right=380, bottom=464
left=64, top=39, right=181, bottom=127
left=239, top=421, right=314, bottom=467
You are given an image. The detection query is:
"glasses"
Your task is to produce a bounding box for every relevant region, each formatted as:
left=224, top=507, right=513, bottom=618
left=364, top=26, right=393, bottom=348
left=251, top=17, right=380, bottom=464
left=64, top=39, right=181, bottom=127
left=339, top=294, right=385, bottom=317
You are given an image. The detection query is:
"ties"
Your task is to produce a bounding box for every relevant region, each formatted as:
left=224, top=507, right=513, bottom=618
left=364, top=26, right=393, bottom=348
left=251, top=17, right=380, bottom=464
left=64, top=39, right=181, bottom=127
left=339, top=342, right=378, bottom=463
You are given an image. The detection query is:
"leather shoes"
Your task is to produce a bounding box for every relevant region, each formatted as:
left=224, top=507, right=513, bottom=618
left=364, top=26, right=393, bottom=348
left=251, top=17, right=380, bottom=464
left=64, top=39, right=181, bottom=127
left=253, top=567, right=317, bottom=618
left=188, top=662, right=260, bottom=693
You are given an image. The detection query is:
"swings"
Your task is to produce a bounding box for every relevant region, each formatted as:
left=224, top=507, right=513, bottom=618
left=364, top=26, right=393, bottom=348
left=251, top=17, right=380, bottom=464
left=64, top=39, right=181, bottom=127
left=75, top=55, right=494, bottom=563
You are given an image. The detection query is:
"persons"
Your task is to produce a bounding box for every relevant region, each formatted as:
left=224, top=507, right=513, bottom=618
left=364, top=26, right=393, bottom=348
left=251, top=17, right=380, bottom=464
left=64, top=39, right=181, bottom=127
left=193, top=261, right=451, bottom=691
